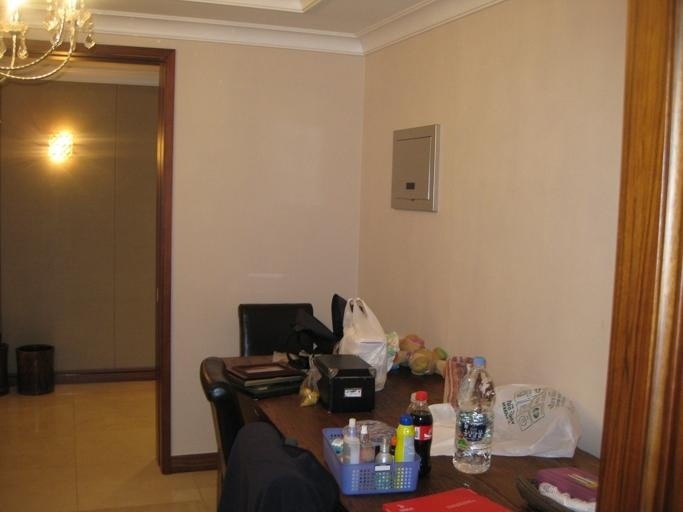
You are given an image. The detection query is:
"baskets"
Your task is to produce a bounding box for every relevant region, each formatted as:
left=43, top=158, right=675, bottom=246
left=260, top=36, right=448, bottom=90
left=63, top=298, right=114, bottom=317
left=321, top=427, right=422, bottom=495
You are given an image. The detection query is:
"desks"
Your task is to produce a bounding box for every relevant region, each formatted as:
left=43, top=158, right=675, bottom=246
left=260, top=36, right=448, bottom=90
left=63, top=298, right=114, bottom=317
left=225, top=354, right=612, bottom=512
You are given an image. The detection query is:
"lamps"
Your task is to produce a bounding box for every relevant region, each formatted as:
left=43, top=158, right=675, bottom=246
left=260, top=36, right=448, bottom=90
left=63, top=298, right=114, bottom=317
left=0, top=2, right=98, bottom=86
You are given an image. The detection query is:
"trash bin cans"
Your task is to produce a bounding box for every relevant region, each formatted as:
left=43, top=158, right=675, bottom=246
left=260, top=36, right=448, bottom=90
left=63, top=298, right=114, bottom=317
left=16, top=344, right=56, bottom=395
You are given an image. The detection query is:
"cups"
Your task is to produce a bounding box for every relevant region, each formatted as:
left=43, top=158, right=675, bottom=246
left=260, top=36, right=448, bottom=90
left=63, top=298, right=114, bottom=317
left=443, top=357, right=476, bottom=410
left=0, top=342, right=57, bottom=396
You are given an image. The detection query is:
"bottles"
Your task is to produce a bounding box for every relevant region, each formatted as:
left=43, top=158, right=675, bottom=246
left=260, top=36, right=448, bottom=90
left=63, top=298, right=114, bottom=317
left=407, top=390, right=433, bottom=475
left=451, top=356, right=495, bottom=476
left=342, top=415, right=415, bottom=490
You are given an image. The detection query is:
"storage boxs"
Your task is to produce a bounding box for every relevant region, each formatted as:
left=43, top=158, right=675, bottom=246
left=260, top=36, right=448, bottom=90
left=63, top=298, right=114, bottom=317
left=313, top=355, right=376, bottom=416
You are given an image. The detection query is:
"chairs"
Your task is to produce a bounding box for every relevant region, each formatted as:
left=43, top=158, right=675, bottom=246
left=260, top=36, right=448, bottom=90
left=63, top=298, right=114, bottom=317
left=234, top=422, right=335, bottom=510
left=199, top=356, right=244, bottom=511
